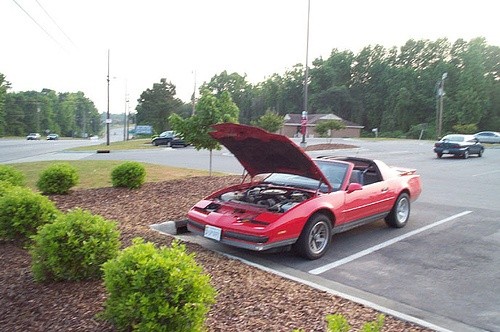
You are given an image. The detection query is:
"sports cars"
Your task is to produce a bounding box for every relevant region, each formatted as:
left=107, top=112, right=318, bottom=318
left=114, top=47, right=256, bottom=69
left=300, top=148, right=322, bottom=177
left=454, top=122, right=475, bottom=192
left=184, top=121, right=423, bottom=262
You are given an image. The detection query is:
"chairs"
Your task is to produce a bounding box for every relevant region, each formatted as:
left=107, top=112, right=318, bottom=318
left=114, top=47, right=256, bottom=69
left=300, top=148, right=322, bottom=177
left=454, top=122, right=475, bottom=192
left=342, top=169, right=365, bottom=186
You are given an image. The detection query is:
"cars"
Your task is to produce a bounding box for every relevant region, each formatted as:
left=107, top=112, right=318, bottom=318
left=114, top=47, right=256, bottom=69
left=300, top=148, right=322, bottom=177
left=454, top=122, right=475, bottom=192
left=474, top=131, right=500, bottom=143
left=27, top=133, right=41, bottom=140
left=136, top=126, right=153, bottom=136
left=151, top=131, right=188, bottom=147
left=433, top=134, right=485, bottom=159
left=47, top=134, right=59, bottom=140
